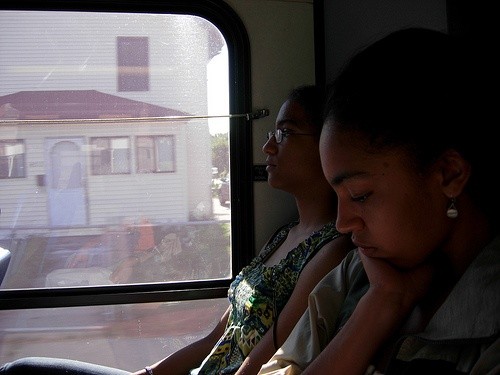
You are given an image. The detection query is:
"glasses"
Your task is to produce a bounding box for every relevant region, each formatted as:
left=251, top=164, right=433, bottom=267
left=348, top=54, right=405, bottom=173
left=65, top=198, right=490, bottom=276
left=267, top=129, right=317, bottom=144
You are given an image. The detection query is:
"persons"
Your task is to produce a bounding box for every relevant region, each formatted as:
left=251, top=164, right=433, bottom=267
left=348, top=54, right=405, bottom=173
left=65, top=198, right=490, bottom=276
left=256, top=29, right=500, bottom=375
left=131, top=86, right=356, bottom=375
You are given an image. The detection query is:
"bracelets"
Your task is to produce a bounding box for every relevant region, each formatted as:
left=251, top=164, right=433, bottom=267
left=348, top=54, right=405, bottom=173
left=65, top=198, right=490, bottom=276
left=144, top=366, right=154, bottom=375
